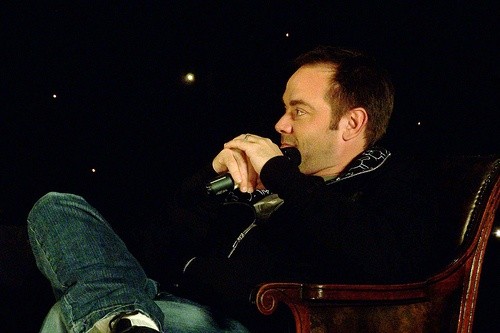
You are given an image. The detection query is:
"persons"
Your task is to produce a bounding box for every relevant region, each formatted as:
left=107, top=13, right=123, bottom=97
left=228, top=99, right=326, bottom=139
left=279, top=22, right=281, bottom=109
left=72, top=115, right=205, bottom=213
left=24, top=44, right=447, bottom=333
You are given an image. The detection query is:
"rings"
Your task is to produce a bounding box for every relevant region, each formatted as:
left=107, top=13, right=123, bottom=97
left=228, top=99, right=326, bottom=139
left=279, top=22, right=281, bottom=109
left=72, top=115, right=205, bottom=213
left=244, top=135, right=252, bottom=141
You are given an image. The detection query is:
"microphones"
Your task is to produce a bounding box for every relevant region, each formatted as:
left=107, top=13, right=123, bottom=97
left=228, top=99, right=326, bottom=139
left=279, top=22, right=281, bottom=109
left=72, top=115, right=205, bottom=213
left=204, top=147, right=302, bottom=195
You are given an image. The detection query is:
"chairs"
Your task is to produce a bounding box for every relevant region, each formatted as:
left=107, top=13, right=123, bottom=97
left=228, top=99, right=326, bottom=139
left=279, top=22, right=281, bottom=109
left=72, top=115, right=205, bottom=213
left=248, top=154, right=500, bottom=333
